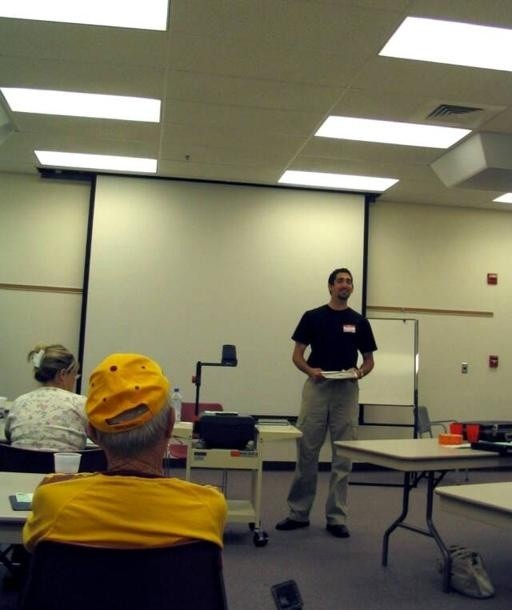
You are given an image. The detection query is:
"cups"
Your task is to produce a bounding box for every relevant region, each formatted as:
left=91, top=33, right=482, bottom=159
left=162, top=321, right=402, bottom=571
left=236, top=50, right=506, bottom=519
left=0, top=396, right=8, bottom=410
left=53, top=452, right=82, bottom=473
left=466, top=424, right=479, bottom=442
left=450, top=422, right=463, bottom=436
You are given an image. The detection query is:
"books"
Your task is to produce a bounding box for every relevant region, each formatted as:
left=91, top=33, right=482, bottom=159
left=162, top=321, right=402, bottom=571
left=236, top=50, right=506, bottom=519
left=258, top=416, right=290, bottom=427
left=204, top=409, right=240, bottom=417
left=471, top=438, right=511, bottom=455
left=317, top=370, right=360, bottom=380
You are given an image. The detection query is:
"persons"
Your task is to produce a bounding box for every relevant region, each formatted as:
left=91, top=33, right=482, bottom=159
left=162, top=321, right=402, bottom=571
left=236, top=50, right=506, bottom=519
left=4, top=344, right=88, bottom=454
left=24, top=354, right=227, bottom=560
left=274, top=269, right=377, bottom=539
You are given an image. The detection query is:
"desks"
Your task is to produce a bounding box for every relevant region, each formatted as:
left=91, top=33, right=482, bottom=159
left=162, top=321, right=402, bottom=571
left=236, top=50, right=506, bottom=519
left=170, top=418, right=303, bottom=498
left=333, top=437, right=512, bottom=593
left=0, top=471, right=47, bottom=524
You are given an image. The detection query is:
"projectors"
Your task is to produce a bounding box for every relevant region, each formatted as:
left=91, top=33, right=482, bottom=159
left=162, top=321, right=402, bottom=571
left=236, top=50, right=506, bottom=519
left=194, top=410, right=256, bottom=449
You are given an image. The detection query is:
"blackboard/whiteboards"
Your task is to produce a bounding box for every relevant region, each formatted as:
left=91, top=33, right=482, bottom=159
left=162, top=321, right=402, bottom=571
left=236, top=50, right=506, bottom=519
left=359, top=318, right=419, bottom=407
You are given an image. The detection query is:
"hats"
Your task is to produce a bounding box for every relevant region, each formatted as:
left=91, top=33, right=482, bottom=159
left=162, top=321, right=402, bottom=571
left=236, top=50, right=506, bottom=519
left=85, top=353, right=171, bottom=434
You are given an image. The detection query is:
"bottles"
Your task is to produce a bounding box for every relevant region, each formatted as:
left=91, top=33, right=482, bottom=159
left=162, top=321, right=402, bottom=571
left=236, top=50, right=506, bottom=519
left=171, top=388, right=182, bottom=422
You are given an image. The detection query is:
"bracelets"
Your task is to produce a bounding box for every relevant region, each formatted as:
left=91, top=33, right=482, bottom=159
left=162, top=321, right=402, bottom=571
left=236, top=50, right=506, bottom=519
left=360, top=366, right=364, bottom=377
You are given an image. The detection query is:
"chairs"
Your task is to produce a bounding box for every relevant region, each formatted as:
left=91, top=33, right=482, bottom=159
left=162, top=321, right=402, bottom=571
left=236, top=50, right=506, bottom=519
left=165, top=403, right=223, bottom=477
left=413, top=407, right=469, bottom=482
left=0, top=442, right=107, bottom=473
left=28, top=539, right=227, bottom=610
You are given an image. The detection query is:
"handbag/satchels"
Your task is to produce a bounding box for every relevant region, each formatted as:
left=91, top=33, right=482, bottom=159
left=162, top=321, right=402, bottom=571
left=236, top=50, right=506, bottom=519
left=436, top=544, right=495, bottom=599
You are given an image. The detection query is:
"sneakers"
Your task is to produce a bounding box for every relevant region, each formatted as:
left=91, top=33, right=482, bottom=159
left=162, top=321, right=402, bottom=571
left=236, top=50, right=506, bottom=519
left=276, top=517, right=310, bottom=530
left=326, top=524, right=349, bottom=537
